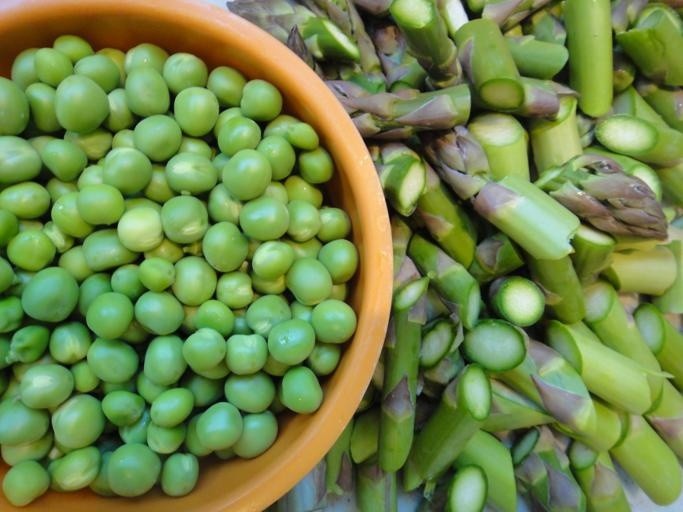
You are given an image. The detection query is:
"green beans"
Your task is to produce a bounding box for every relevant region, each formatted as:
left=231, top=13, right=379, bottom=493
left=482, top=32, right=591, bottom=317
left=0, top=35, right=361, bottom=504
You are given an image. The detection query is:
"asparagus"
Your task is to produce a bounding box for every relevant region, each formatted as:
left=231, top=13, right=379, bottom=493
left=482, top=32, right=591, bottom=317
left=228, top=0, right=683, bottom=512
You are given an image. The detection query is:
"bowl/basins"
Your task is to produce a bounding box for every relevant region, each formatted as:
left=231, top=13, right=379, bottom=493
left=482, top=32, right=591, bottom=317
left=0, top=0, right=397, bottom=512
